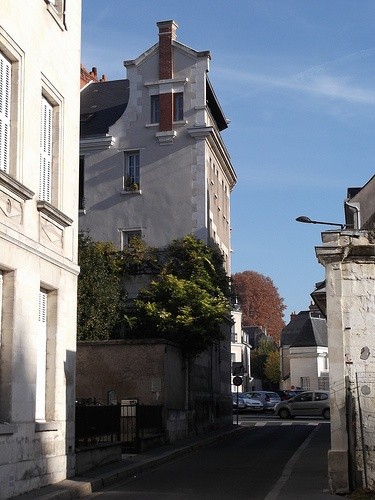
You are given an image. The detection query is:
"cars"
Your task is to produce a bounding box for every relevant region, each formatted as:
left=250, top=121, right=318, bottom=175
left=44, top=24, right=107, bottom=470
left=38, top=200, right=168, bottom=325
left=245, top=391, right=281, bottom=412
left=238, top=392, right=264, bottom=412
left=272, top=389, right=330, bottom=422
left=230, top=393, right=246, bottom=412
